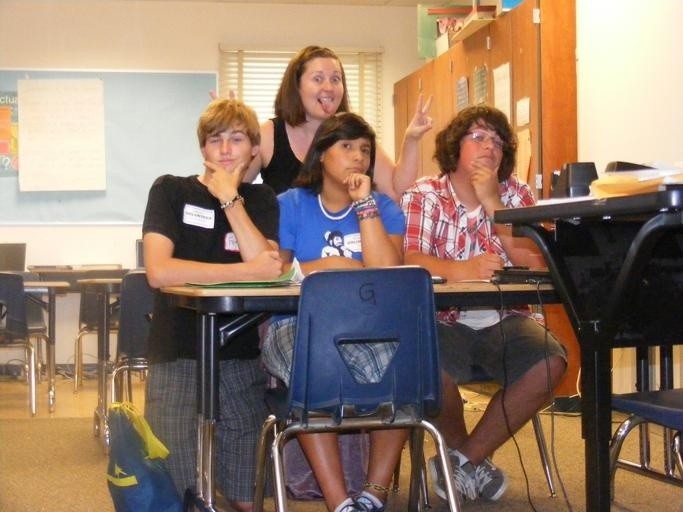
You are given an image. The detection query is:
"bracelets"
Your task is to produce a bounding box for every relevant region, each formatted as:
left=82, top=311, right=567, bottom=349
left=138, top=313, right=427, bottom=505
left=351, top=194, right=382, bottom=220
left=220, top=194, right=245, bottom=210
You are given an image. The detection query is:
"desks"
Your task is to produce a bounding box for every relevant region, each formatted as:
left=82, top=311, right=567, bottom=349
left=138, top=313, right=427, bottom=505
left=494, top=190, right=683, bottom=512
left=2, top=267, right=143, bottom=411
left=162, top=279, right=676, bottom=512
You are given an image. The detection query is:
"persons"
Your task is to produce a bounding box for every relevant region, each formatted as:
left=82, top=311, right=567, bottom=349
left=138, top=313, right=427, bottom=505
left=402, top=107, right=568, bottom=496
left=209, top=44, right=437, bottom=198
left=138, top=98, right=281, bottom=512
left=277, top=113, right=408, bottom=511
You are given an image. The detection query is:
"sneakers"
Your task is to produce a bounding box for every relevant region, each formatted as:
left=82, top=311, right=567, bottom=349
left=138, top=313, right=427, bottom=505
left=427, top=452, right=477, bottom=504
left=473, top=460, right=509, bottom=504
left=357, top=495, right=378, bottom=512
left=332, top=500, right=353, bottom=510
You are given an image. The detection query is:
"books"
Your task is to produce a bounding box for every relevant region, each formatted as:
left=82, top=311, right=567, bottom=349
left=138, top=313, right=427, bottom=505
left=187, top=273, right=298, bottom=288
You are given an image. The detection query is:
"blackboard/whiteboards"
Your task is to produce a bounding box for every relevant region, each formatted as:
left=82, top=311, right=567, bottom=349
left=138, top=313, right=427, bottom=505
left=1, top=66, right=219, bottom=226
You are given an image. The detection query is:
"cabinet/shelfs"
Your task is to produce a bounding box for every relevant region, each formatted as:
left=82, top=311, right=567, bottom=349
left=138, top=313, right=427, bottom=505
left=393, top=0, right=576, bottom=401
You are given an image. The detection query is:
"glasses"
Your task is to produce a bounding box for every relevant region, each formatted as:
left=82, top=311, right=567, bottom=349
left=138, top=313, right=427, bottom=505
left=459, top=130, right=507, bottom=148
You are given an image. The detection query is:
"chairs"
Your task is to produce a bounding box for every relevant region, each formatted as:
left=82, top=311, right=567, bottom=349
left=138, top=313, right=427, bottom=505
left=74, top=288, right=131, bottom=393
left=253, top=269, right=462, bottom=512
left=115, top=270, right=154, bottom=410
left=0, top=272, right=38, bottom=415
left=0, top=244, right=52, bottom=391
left=610, top=386, right=683, bottom=512
